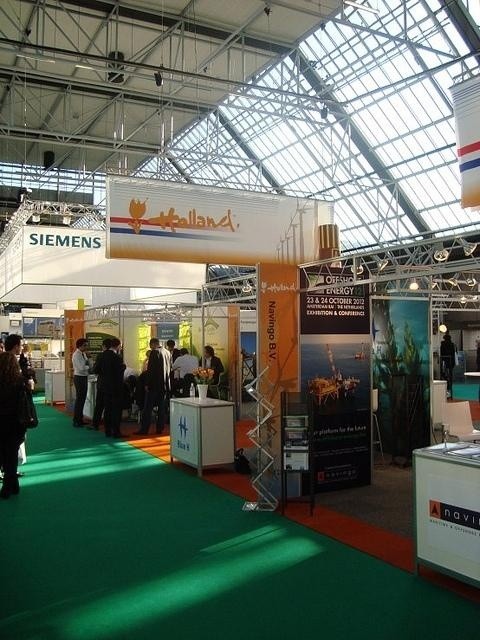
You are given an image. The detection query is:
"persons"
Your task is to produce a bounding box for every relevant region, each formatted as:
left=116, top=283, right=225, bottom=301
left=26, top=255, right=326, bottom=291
left=439, top=333, right=458, bottom=392
left=474, top=338, right=480, bottom=372
left=72, top=338, right=224, bottom=440
left=0, top=334, right=40, bottom=501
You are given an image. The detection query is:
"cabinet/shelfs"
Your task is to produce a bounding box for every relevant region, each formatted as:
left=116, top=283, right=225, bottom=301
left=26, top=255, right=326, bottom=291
left=411, top=442, right=480, bottom=588
left=169, top=397, right=237, bottom=478
left=434, top=380, right=446, bottom=431
left=44, top=370, right=65, bottom=407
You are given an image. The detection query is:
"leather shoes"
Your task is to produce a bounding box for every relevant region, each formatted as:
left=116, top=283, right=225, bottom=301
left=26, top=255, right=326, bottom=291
left=74, top=423, right=164, bottom=439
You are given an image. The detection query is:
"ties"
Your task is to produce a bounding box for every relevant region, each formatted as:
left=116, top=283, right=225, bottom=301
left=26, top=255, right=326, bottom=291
left=82, top=354, right=85, bottom=359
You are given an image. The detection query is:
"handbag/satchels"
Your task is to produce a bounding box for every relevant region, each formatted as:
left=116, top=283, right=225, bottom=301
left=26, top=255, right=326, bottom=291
left=234, top=448, right=252, bottom=475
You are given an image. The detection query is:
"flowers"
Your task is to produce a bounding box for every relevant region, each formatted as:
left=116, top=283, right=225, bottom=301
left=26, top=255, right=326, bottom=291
left=193, top=367, right=215, bottom=384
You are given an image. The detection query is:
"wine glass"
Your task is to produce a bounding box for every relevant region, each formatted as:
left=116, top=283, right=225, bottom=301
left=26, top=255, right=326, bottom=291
left=442, top=424, right=450, bottom=450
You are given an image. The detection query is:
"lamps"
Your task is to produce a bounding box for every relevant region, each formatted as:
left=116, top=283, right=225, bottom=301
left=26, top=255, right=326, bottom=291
left=350, top=237, right=479, bottom=275
left=242, top=279, right=252, bottom=293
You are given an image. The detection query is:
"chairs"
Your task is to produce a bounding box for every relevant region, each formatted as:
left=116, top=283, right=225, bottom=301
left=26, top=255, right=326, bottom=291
left=440, top=399, right=480, bottom=445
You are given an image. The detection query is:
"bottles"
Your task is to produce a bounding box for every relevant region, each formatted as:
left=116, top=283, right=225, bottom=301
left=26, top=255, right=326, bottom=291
left=189, top=383, right=195, bottom=402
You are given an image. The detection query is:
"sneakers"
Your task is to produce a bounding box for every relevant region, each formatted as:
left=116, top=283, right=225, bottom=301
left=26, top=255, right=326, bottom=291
left=17, top=472, right=24, bottom=477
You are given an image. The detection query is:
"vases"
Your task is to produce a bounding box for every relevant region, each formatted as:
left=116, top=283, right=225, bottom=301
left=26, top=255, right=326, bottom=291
left=197, top=385, right=208, bottom=400
